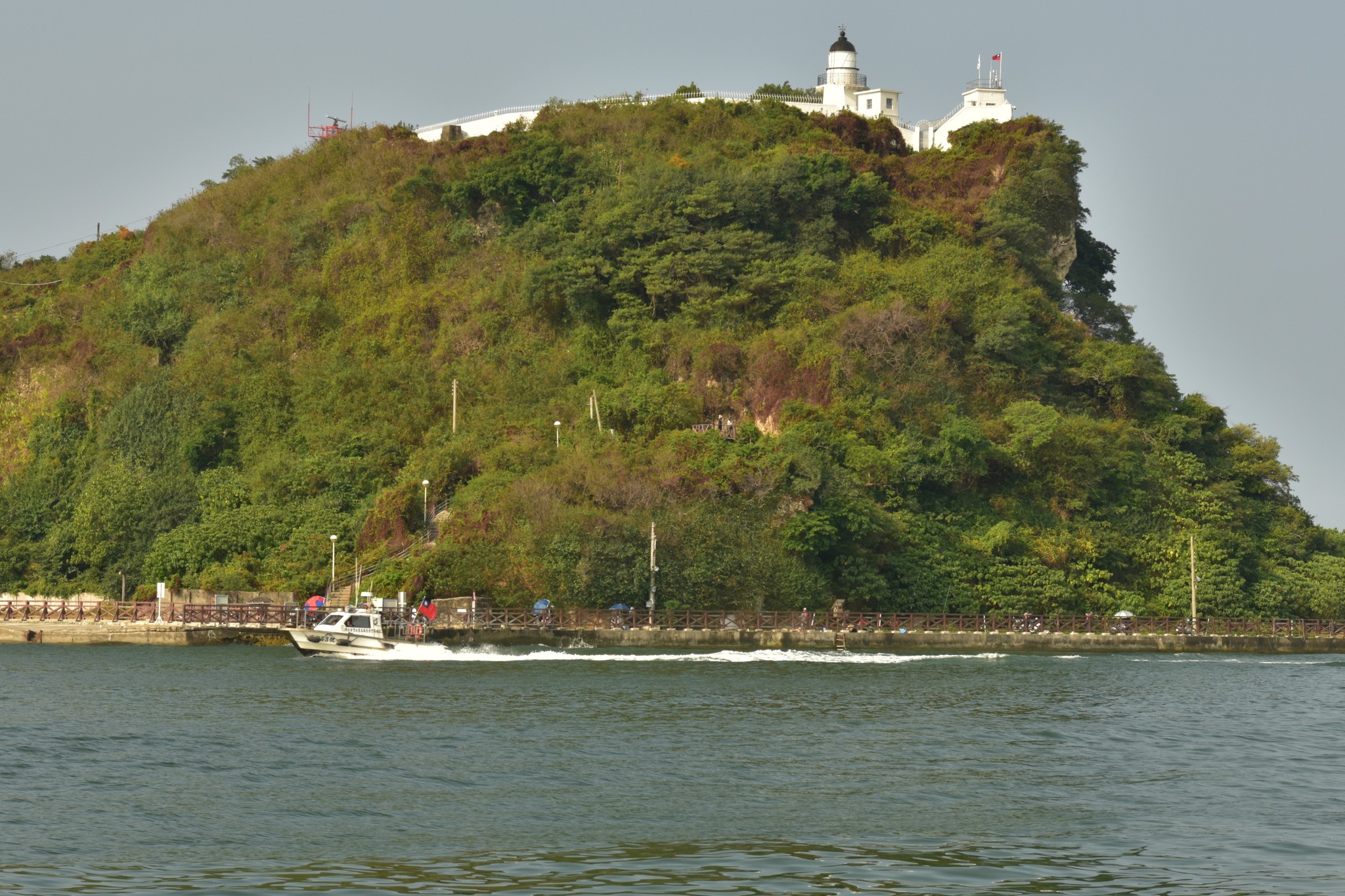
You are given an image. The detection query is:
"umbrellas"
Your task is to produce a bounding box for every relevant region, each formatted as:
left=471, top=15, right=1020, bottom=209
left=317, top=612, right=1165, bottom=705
left=531, top=599, right=550, bottom=615
left=304, top=596, right=325, bottom=610
left=1114, top=610, right=1133, bottom=618
left=609, top=603, right=630, bottom=615
left=246, top=596, right=274, bottom=604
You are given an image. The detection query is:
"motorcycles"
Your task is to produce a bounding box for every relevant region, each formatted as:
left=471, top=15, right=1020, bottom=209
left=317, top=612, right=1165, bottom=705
left=1107, top=621, right=1126, bottom=635
left=1176, top=618, right=1193, bottom=634
left=533, top=616, right=552, bottom=629
left=1028, top=618, right=1042, bottom=634
left=1011, top=617, right=1025, bottom=632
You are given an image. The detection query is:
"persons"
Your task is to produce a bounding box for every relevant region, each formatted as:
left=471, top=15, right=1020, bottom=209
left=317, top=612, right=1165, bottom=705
left=629, top=607, right=635, bottom=628
left=332, top=620, right=337, bottom=625
left=1123, top=618, right=1131, bottom=635
left=316, top=608, right=323, bottom=619
left=1085, top=611, right=1099, bottom=628
left=550, top=605, right=554, bottom=618
left=719, top=415, right=724, bottom=435
left=411, top=605, right=417, bottom=623
left=727, top=417, right=734, bottom=437
left=801, top=608, right=808, bottom=627
left=347, top=621, right=352, bottom=626
left=859, top=619, right=866, bottom=630
left=1023, top=611, right=1032, bottom=628
left=1185, top=618, right=1192, bottom=631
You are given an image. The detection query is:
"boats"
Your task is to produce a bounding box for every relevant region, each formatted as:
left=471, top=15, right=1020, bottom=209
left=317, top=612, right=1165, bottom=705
left=280, top=558, right=453, bottom=658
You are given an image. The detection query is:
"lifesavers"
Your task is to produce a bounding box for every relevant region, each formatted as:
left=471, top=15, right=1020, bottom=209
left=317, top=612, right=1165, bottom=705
left=405, top=624, right=413, bottom=638
left=413, top=624, right=423, bottom=638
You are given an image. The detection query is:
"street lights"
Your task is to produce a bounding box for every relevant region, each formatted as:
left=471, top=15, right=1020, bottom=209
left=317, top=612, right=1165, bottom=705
left=554, top=421, right=561, bottom=456
left=646, top=566, right=659, bottom=624
left=422, top=480, right=430, bottom=529
left=330, top=535, right=338, bottom=592
left=118, top=570, right=127, bottom=620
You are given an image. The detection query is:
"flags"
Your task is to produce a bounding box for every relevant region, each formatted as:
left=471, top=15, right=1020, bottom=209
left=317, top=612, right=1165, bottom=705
left=977, top=56, right=980, bottom=69
left=418, top=599, right=438, bottom=620
left=992, top=54, right=1000, bottom=60
